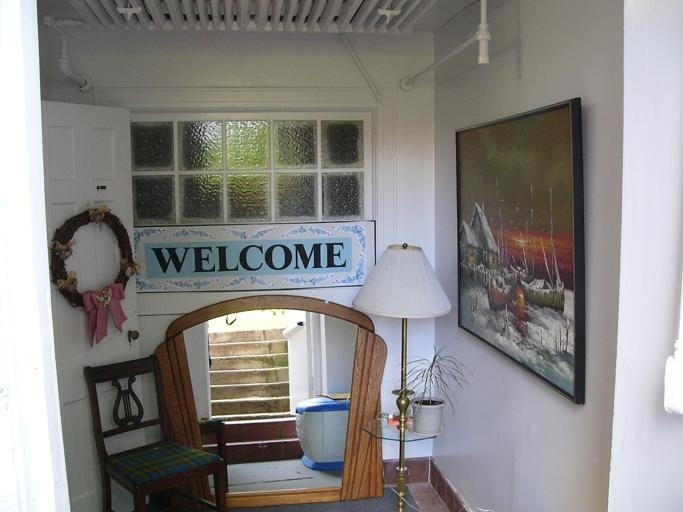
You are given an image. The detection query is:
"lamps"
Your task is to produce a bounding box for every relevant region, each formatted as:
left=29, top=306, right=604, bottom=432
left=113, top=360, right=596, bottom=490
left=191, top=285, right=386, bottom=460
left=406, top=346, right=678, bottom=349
left=352, top=243, right=452, bottom=512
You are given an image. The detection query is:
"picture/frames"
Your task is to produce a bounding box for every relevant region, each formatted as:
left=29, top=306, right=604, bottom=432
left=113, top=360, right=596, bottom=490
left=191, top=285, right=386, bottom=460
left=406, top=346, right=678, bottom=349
left=454, top=97, right=586, bottom=405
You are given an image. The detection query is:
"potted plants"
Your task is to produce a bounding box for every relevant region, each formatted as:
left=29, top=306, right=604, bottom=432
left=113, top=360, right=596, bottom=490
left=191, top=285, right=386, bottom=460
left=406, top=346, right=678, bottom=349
left=407, top=338, right=469, bottom=436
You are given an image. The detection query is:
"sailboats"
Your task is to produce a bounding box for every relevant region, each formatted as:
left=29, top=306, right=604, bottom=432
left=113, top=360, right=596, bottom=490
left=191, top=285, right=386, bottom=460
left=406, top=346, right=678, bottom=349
left=484, top=178, right=564, bottom=311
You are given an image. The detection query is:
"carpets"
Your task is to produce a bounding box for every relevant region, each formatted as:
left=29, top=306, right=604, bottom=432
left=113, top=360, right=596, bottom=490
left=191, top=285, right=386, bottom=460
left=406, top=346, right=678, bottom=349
left=228, top=486, right=419, bottom=512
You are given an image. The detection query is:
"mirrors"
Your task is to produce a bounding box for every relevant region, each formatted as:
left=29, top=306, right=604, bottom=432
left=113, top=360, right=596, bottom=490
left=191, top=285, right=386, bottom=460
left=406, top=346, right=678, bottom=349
left=153, top=295, right=387, bottom=509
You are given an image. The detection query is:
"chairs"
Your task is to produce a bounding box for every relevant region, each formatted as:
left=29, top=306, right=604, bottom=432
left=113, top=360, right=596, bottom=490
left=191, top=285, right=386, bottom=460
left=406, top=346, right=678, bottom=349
left=83, top=354, right=227, bottom=512
left=198, top=418, right=229, bottom=493
